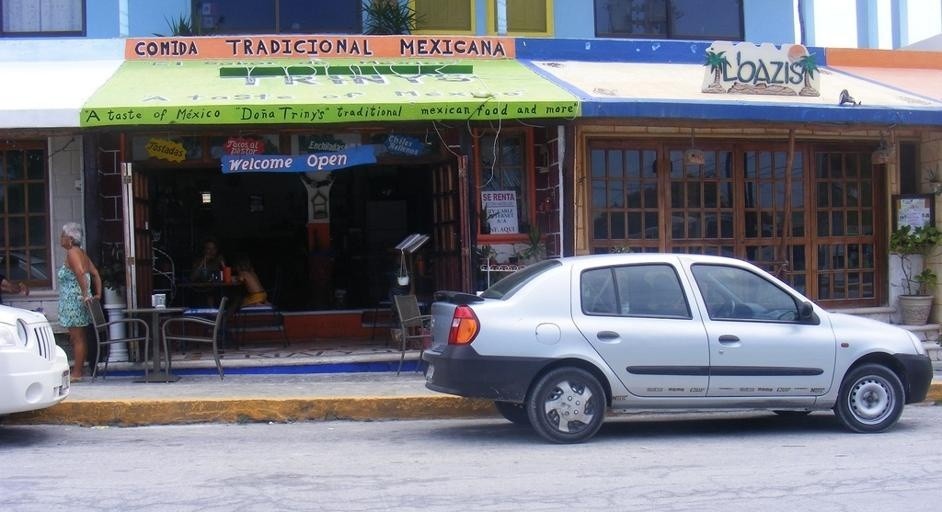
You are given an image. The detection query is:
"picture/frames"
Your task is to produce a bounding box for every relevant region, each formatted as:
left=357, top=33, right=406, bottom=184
left=892, top=192, right=935, bottom=239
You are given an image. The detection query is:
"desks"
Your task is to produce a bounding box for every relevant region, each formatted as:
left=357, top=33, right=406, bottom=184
left=176, top=278, right=244, bottom=352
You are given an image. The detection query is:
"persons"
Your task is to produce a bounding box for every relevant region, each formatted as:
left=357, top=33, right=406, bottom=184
left=0, top=272, right=29, bottom=302
left=57, top=222, right=103, bottom=383
left=192, top=240, right=226, bottom=306
left=235, top=259, right=272, bottom=307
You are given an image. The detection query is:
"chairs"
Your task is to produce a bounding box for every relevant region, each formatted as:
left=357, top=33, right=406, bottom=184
left=391, top=293, right=432, bottom=378
left=233, top=267, right=290, bottom=349
left=86, top=295, right=235, bottom=382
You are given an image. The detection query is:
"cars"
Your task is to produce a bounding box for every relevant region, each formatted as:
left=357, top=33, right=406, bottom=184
left=0, top=303, right=76, bottom=428
left=417, top=248, right=935, bottom=447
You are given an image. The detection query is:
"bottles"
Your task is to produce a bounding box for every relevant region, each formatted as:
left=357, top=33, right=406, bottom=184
left=201, top=268, right=208, bottom=282
left=223, top=266, right=232, bottom=284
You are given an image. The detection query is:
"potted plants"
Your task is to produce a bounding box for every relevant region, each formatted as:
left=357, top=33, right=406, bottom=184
left=889, top=222, right=941, bottom=326
left=477, top=200, right=500, bottom=233
left=392, top=267, right=415, bottom=287
